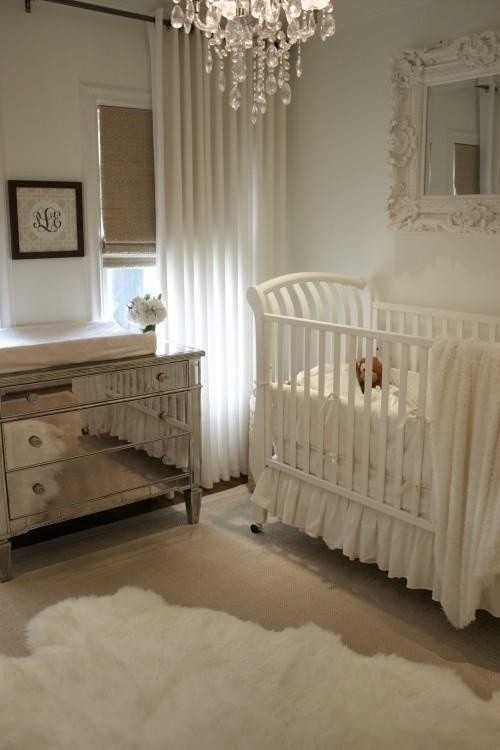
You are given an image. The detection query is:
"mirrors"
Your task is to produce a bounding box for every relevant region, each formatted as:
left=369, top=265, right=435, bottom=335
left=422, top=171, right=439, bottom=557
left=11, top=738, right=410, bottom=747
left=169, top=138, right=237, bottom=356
left=386, top=32, right=499, bottom=230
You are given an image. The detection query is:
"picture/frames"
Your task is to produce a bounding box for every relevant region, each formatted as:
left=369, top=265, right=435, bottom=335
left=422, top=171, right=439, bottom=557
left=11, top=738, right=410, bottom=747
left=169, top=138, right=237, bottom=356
left=8, top=179, right=85, bottom=261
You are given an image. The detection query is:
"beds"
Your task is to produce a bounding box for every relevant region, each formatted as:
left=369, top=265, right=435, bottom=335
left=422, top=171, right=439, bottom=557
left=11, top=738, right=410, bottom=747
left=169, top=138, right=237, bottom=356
left=246, top=270, right=498, bottom=620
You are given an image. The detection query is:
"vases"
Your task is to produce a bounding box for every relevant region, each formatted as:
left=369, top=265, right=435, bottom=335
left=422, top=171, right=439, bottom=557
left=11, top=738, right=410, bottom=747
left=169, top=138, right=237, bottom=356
left=141, top=323, right=155, bottom=333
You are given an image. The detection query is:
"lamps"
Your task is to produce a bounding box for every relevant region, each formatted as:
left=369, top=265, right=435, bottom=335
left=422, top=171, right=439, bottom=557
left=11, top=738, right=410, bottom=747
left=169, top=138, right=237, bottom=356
left=169, top=0, right=336, bottom=124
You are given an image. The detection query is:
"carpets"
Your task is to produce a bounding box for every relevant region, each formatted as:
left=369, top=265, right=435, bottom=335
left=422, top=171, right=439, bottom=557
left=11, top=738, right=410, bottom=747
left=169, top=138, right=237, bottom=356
left=0, top=585, right=498, bottom=748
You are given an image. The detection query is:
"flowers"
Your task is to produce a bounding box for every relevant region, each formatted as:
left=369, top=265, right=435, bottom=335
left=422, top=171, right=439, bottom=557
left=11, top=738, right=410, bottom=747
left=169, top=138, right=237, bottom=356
left=127, top=294, right=167, bottom=328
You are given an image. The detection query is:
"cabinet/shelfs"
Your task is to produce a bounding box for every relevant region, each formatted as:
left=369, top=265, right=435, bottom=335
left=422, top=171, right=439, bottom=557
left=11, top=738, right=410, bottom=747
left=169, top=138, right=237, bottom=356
left=2, top=338, right=206, bottom=584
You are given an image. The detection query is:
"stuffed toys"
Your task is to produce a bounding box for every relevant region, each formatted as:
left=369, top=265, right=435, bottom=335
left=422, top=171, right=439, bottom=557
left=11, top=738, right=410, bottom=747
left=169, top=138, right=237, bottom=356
left=356, top=357, right=382, bottom=394
